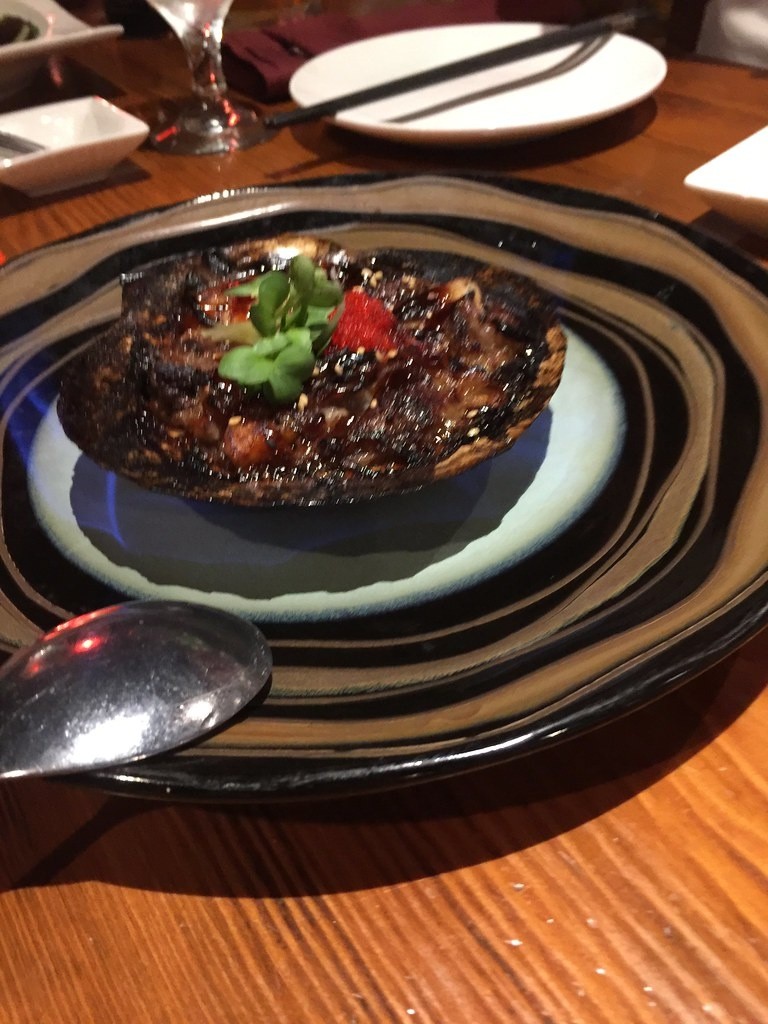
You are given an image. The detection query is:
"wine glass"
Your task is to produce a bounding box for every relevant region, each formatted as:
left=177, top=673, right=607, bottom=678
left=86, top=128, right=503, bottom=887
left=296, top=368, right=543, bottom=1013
left=122, top=0, right=268, bottom=156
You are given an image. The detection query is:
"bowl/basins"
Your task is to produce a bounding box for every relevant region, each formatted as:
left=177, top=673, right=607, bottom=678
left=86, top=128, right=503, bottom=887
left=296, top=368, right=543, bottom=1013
left=0, top=0, right=124, bottom=102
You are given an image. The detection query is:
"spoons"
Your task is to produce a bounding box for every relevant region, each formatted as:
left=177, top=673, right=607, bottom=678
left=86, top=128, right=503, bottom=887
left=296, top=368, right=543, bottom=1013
left=0, top=597, right=276, bottom=787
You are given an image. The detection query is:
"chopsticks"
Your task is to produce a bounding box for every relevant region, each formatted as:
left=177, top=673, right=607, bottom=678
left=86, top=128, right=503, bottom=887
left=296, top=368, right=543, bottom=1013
left=261, top=3, right=659, bottom=127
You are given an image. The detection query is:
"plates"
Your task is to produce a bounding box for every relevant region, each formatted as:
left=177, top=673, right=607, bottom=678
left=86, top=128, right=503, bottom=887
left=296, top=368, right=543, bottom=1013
left=0, top=94, right=151, bottom=199
left=54, top=227, right=567, bottom=513
left=288, top=22, right=668, bottom=149
left=0, top=169, right=768, bottom=804
left=683, top=124, right=768, bottom=241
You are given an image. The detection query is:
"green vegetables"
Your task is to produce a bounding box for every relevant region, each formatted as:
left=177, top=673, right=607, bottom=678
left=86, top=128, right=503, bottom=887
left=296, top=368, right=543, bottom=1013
left=195, top=252, right=347, bottom=401
